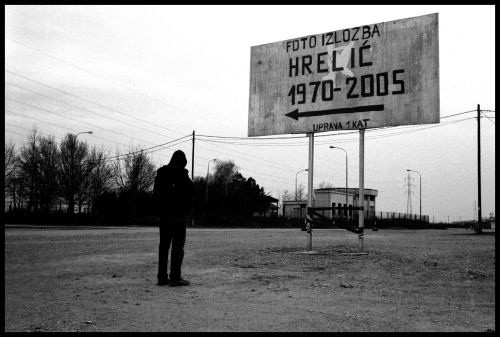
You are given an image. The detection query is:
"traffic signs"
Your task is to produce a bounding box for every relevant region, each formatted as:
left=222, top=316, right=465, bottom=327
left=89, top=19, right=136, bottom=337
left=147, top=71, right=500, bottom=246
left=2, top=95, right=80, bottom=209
left=247, top=12, right=440, bottom=139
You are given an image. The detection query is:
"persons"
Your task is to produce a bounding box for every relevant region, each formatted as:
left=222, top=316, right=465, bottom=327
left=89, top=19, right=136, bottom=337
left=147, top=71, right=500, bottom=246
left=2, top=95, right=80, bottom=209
left=152, top=150, right=197, bottom=286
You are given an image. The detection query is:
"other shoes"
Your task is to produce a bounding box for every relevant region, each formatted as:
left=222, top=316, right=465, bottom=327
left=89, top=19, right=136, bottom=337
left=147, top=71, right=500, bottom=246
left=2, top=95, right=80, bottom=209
left=157, top=278, right=171, bottom=285
left=171, top=278, right=190, bottom=286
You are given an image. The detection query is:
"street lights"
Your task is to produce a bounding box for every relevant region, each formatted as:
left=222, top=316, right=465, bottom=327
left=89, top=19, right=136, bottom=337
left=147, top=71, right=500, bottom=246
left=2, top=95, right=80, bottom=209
left=329, top=145, right=349, bottom=228
left=408, top=169, right=422, bottom=228
left=294, top=169, right=309, bottom=200
left=71, top=130, right=94, bottom=170
